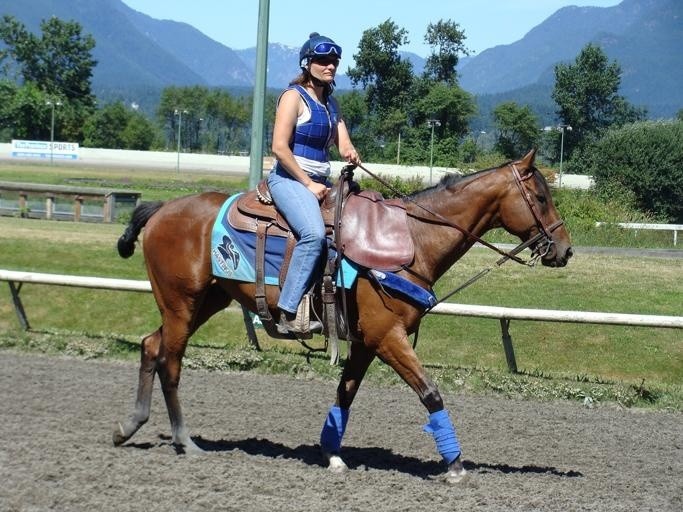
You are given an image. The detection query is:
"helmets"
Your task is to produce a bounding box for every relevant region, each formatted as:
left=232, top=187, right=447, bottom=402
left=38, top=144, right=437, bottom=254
left=298, top=32, right=341, bottom=70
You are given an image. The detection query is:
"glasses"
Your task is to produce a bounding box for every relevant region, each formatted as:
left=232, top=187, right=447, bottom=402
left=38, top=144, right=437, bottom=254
left=301, top=43, right=341, bottom=59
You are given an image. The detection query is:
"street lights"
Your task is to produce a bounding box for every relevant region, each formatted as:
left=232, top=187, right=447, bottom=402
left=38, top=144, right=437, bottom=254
left=173, top=110, right=188, bottom=173
left=539, top=123, right=572, bottom=188
left=44, top=102, right=62, bottom=163
left=425, top=119, right=441, bottom=186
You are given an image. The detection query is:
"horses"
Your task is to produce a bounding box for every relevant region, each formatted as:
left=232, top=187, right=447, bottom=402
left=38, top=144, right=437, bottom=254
left=110, top=147, right=575, bottom=482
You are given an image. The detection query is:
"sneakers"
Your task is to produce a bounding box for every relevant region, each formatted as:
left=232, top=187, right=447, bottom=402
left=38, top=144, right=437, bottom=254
left=276, top=310, right=322, bottom=335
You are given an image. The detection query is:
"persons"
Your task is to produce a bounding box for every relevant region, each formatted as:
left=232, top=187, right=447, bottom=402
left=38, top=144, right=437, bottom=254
left=265, top=32, right=363, bottom=335
